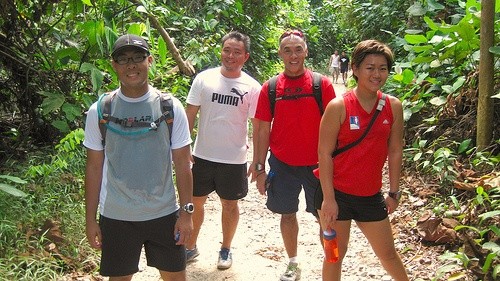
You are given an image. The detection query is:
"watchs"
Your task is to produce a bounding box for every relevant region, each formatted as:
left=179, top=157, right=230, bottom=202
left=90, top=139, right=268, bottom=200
left=387, top=190, right=402, bottom=200
left=179, top=203, right=194, bottom=213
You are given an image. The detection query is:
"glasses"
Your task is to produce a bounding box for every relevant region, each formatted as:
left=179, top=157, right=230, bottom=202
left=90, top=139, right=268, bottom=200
left=114, top=55, right=150, bottom=65
left=278, top=30, right=307, bottom=46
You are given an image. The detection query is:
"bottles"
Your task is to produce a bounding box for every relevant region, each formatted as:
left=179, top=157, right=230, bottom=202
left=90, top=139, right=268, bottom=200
left=322, top=229, right=339, bottom=263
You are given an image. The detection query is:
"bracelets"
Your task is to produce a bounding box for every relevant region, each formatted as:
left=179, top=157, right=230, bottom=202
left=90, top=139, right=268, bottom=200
left=254, top=163, right=265, bottom=171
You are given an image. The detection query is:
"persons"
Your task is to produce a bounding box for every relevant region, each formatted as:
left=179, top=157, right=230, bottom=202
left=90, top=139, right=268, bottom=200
left=329, top=50, right=340, bottom=84
left=338, top=51, right=350, bottom=85
left=256, top=31, right=336, bottom=281
left=178, top=31, right=262, bottom=269
left=84, top=34, right=194, bottom=281
left=312, top=39, right=408, bottom=281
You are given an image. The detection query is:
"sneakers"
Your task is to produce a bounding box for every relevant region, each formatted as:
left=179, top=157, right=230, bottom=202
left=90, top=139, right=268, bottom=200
left=184, top=243, right=199, bottom=262
left=279, top=261, right=301, bottom=281
left=216, top=247, right=232, bottom=268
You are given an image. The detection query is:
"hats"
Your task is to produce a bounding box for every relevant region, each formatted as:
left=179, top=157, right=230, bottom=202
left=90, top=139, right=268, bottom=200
left=110, top=35, right=151, bottom=58
